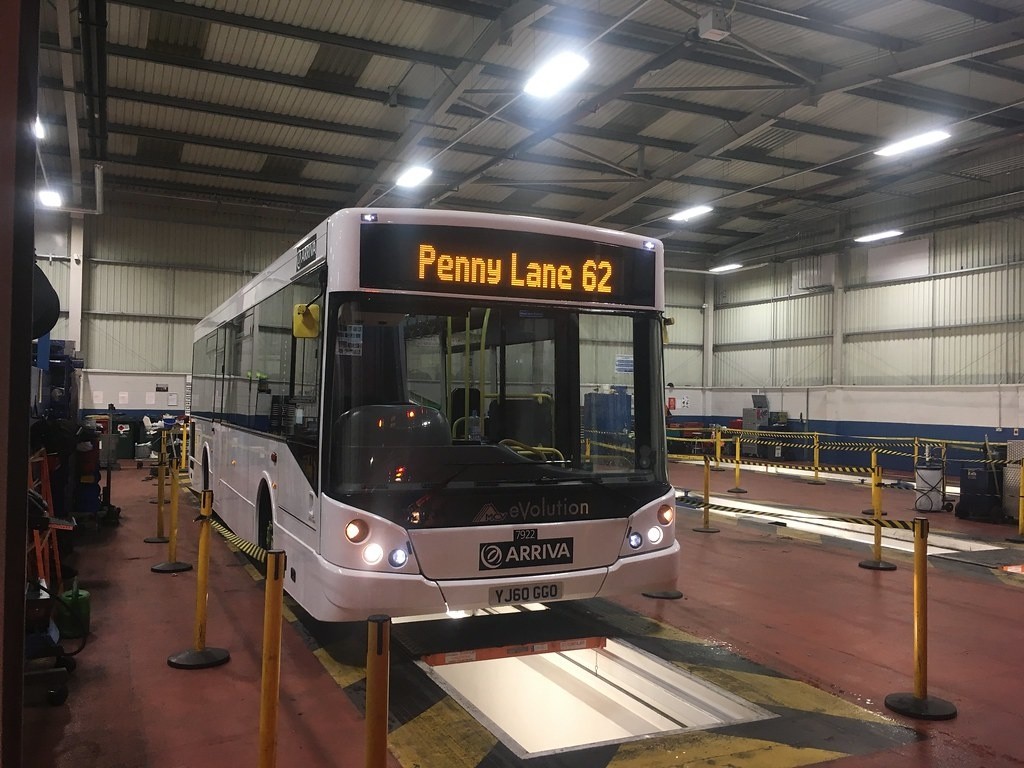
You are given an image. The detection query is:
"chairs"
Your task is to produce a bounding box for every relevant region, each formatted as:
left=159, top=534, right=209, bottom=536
left=451, top=388, right=552, bottom=446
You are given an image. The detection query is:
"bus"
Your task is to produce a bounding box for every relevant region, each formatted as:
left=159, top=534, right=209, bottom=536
left=188, top=208, right=680, bottom=622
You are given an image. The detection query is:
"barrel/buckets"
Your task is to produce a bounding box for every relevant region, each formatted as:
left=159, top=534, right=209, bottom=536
left=916, top=466, right=942, bottom=511
left=135, top=445, right=150, bottom=458
left=163, top=419, right=175, bottom=429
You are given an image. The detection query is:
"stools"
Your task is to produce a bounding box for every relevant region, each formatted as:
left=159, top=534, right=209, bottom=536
left=692, top=432, right=702, bottom=454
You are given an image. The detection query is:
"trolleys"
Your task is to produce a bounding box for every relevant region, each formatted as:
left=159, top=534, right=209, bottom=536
left=915, top=447, right=954, bottom=513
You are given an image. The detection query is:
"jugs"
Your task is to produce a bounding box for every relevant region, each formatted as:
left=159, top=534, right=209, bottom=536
left=52, top=577, right=90, bottom=636
